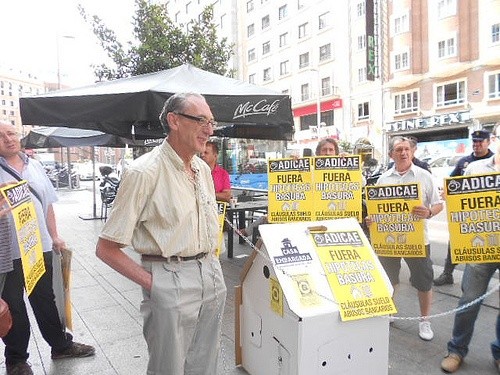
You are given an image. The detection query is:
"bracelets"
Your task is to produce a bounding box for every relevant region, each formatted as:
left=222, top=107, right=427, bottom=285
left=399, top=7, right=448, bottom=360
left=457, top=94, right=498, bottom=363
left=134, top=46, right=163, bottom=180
left=430, top=210, right=432, bottom=216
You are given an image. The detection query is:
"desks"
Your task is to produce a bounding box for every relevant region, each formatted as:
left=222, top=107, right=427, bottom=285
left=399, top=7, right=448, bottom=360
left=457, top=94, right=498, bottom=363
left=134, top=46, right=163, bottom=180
left=225, top=200, right=269, bottom=260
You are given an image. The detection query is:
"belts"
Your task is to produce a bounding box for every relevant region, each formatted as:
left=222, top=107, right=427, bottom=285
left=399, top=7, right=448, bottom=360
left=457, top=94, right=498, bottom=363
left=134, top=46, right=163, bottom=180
left=142, top=251, right=208, bottom=261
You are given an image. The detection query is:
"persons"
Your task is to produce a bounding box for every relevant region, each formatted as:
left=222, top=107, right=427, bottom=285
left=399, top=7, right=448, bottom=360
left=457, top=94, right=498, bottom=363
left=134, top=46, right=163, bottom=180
left=0, top=121, right=98, bottom=375
left=292, top=138, right=366, bottom=186
left=438, top=120, right=500, bottom=373
left=235, top=216, right=267, bottom=237
left=434, top=130, right=495, bottom=286
left=200, top=142, right=231, bottom=201
left=95, top=94, right=227, bottom=374
left=365, top=136, right=444, bottom=340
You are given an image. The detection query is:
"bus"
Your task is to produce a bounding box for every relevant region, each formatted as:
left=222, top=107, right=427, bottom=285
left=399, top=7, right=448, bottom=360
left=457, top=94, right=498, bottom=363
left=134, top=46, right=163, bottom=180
left=144, top=136, right=289, bottom=204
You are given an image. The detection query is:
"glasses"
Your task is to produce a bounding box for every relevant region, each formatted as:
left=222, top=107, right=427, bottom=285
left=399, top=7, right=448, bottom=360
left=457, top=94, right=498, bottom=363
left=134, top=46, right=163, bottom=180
left=174, top=113, right=217, bottom=129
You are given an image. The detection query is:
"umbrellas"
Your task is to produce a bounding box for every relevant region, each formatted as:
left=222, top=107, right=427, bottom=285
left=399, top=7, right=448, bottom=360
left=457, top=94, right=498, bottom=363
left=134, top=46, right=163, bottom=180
left=19, top=63, right=294, bottom=141
left=19, top=126, right=164, bottom=220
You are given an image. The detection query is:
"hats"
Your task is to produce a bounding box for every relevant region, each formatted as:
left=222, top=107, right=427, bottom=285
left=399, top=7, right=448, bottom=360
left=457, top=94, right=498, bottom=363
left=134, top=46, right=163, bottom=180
left=471, top=130, right=490, bottom=141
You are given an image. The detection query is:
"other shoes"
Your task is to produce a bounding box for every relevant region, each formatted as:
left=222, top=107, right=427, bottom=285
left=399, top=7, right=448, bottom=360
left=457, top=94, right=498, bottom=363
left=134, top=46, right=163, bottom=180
left=441, top=354, right=463, bottom=373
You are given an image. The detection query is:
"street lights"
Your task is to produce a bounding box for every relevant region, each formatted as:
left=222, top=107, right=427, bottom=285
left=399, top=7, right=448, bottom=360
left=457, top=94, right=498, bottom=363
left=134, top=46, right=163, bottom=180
left=310, top=66, right=321, bottom=139
left=55, top=35, right=77, bottom=168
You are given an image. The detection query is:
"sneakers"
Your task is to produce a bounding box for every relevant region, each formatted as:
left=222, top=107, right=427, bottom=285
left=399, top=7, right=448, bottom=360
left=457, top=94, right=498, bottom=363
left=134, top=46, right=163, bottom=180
left=51, top=341, right=95, bottom=358
left=433, top=273, right=453, bottom=285
left=419, top=321, right=434, bottom=340
left=6, top=361, right=33, bottom=375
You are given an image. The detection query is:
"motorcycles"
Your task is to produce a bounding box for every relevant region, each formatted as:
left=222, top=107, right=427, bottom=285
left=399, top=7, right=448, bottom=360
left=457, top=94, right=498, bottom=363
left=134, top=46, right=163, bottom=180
left=99, top=166, right=121, bottom=207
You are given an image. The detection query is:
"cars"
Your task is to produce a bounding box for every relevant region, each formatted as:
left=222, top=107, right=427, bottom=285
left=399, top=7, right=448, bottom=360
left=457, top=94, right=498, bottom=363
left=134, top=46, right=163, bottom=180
left=39, top=160, right=100, bottom=187
